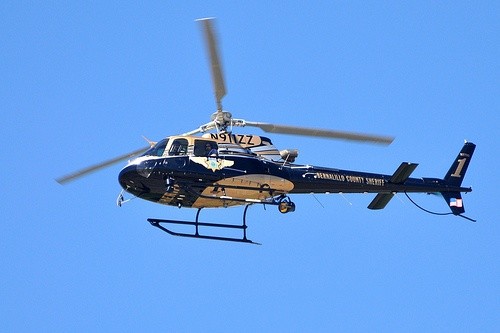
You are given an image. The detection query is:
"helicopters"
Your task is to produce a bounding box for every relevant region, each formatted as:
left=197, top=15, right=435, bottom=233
left=56, top=17, right=476, bottom=247
